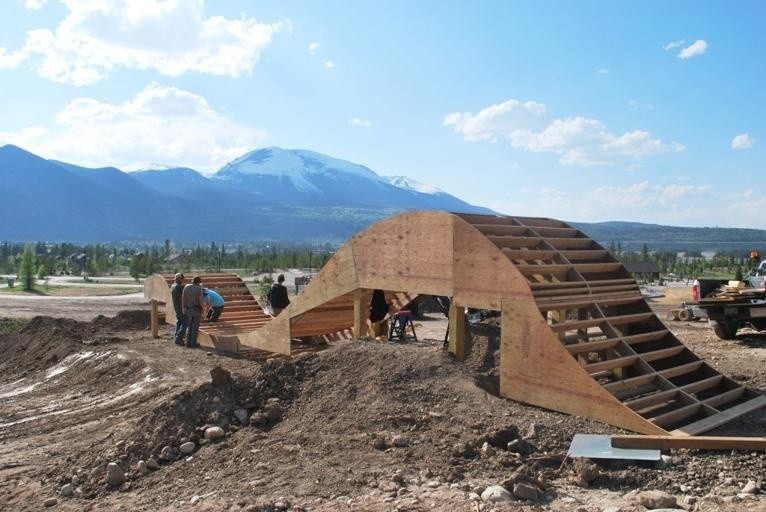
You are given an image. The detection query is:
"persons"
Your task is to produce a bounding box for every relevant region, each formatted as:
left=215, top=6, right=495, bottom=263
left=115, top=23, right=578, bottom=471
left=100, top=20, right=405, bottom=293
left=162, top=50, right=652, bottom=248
left=171, top=273, right=187, bottom=344
left=267, top=275, right=290, bottom=317
left=203, top=287, right=224, bottom=322
left=182, top=277, right=205, bottom=348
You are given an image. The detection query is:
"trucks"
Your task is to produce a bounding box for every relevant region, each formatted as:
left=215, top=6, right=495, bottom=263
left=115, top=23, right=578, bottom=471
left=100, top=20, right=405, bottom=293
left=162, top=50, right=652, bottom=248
left=684, top=259, right=766, bottom=339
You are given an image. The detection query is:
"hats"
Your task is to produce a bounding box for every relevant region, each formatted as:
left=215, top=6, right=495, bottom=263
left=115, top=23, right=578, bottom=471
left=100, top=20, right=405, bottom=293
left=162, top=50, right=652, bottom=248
left=175, top=273, right=184, bottom=280
left=278, top=274, right=285, bottom=281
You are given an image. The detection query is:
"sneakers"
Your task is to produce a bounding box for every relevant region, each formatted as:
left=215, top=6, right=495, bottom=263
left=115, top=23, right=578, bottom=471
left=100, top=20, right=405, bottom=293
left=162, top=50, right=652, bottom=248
left=175, top=339, right=200, bottom=348
